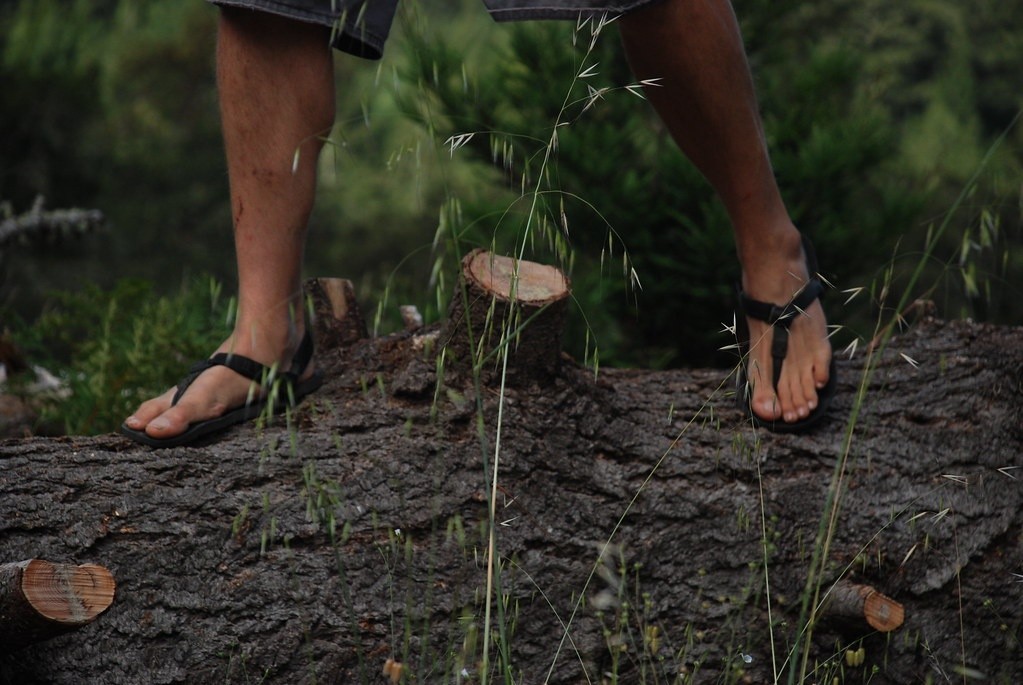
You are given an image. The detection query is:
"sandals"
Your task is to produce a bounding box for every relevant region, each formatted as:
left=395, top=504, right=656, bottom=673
left=736, top=236, right=839, bottom=430
left=122, top=324, right=325, bottom=448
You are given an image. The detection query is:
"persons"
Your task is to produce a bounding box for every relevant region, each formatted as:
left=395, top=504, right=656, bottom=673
left=114, top=0, right=836, bottom=449
